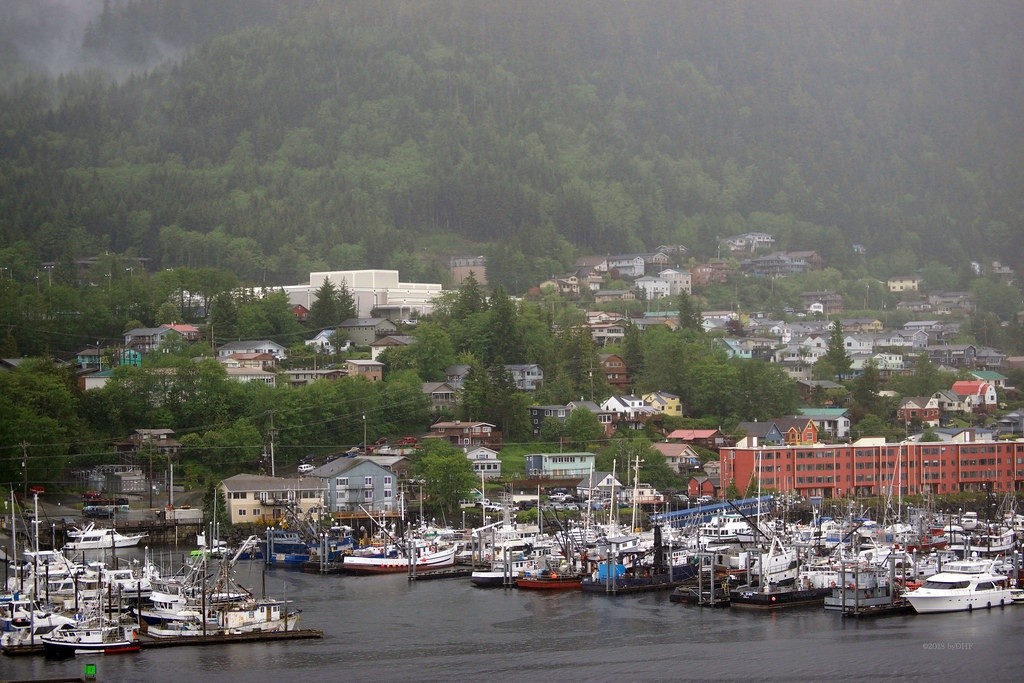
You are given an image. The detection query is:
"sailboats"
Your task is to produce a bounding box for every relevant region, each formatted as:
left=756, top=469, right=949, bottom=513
left=341, top=443, right=1024, bottom=614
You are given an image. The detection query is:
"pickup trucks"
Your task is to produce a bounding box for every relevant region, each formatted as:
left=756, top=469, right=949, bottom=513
left=546, top=502, right=564, bottom=512
left=548, top=492, right=572, bottom=502
left=690, top=499, right=706, bottom=505
left=411, top=444, right=422, bottom=449
left=19, top=509, right=35, bottom=519
left=475, top=498, right=497, bottom=508
left=484, top=502, right=519, bottom=514
left=566, top=496, right=588, bottom=503
left=58, top=516, right=76, bottom=527
left=397, top=437, right=417, bottom=447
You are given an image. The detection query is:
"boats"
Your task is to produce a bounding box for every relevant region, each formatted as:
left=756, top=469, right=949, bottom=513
left=61, top=522, right=150, bottom=550
left=0, top=487, right=305, bottom=657
left=899, top=534, right=1013, bottom=614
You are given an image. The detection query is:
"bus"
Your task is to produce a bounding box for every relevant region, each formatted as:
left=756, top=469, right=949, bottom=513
left=82, top=498, right=130, bottom=516
left=166, top=483, right=192, bottom=492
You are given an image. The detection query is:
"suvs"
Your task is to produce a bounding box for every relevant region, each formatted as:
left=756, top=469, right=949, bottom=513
left=27, top=519, right=43, bottom=527
left=673, top=495, right=689, bottom=502
left=82, top=490, right=101, bottom=498
left=298, top=465, right=315, bottom=474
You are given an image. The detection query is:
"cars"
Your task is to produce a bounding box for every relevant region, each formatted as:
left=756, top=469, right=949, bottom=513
left=381, top=446, right=391, bottom=450
left=577, top=496, right=628, bottom=512
left=297, top=454, right=314, bottom=463
left=323, top=437, right=388, bottom=464
left=563, top=503, right=580, bottom=511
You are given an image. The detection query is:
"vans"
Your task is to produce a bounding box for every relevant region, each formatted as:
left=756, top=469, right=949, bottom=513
left=701, top=495, right=712, bottom=503
